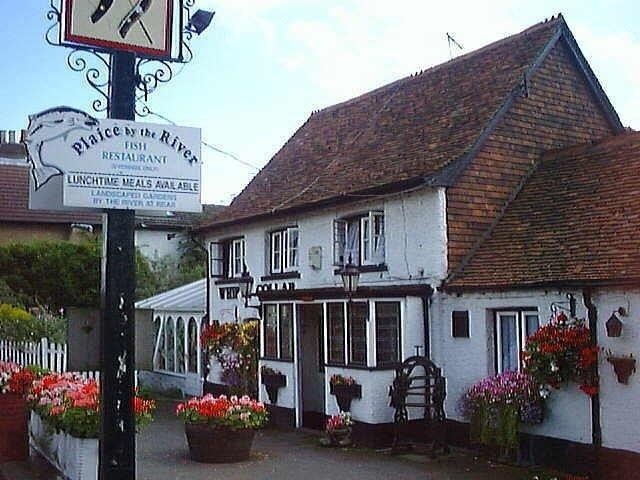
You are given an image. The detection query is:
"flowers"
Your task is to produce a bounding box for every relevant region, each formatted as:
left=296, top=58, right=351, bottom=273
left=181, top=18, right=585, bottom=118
left=324, top=410, right=355, bottom=430
left=521, top=316, right=600, bottom=390
left=203, top=317, right=260, bottom=390
left=24, top=365, right=157, bottom=445
left=175, top=392, right=271, bottom=430
left=453, top=367, right=544, bottom=440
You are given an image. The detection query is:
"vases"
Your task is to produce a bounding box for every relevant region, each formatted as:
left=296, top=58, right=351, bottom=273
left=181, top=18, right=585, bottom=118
left=185, top=418, right=256, bottom=462
left=328, top=427, right=352, bottom=447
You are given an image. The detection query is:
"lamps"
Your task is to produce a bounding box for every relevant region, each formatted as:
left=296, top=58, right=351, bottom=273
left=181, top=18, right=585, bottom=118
left=605, top=308, right=626, bottom=338
left=341, top=251, right=361, bottom=305
left=239, top=262, right=262, bottom=308
